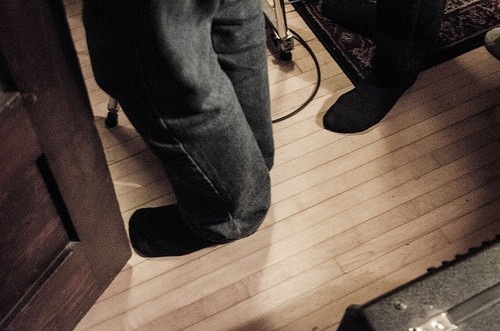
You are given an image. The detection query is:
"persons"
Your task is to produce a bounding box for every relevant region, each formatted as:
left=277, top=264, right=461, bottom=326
left=83, top=0, right=276, bottom=260
